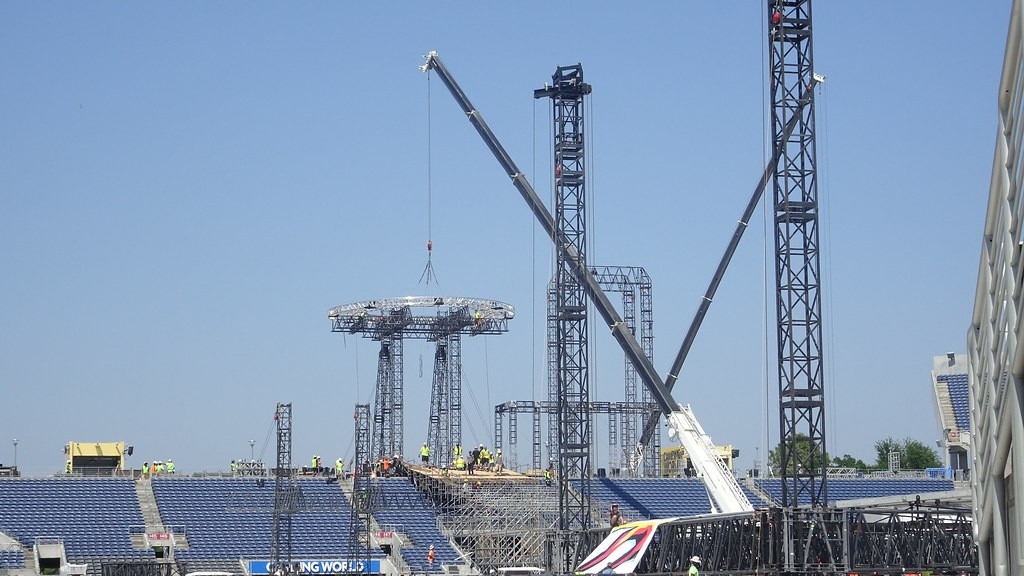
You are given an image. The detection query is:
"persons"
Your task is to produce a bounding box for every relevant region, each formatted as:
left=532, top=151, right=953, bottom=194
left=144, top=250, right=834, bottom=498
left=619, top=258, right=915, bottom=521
left=467, top=449, right=474, bottom=475
left=494, top=448, right=504, bottom=476
left=608, top=502, right=624, bottom=528
left=141, top=462, right=150, bottom=475
left=686, top=555, right=701, bottom=576
left=601, top=561, right=616, bottom=574
left=428, top=544, right=435, bottom=566
left=418, top=443, right=431, bottom=464
left=473, top=444, right=494, bottom=472
left=152, top=460, right=177, bottom=475
left=311, top=455, right=321, bottom=475
left=334, top=456, right=346, bottom=479
left=383, top=455, right=401, bottom=476
left=229, top=459, right=236, bottom=477
left=63, top=459, right=72, bottom=476
left=452, top=442, right=466, bottom=470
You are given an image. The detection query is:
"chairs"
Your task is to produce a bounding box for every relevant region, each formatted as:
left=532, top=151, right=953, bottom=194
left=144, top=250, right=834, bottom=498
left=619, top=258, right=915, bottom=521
left=152, top=487, right=161, bottom=492
left=156, top=478, right=465, bottom=576
left=568, top=478, right=955, bottom=524
left=151, top=478, right=157, bottom=482
left=0, top=478, right=156, bottom=576
left=937, top=372, right=1008, bottom=432
left=154, top=494, right=162, bottom=499
left=153, top=490, right=161, bottom=495
left=151, top=481, right=159, bottom=486
left=152, top=484, right=159, bottom=488
left=155, top=497, right=165, bottom=501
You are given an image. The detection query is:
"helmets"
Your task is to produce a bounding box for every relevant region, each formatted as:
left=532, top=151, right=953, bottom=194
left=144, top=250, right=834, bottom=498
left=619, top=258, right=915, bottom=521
left=430, top=545, right=434, bottom=549
left=339, top=458, right=343, bottom=461
left=238, top=460, right=242, bottom=463
left=490, top=452, right=492, bottom=455
left=313, top=455, right=317, bottom=458
left=144, top=461, right=148, bottom=465
left=317, top=456, right=320, bottom=459
left=251, top=460, right=255, bottom=463
left=469, top=450, right=471, bottom=453
left=153, top=461, right=162, bottom=464
left=168, top=459, right=172, bottom=462
left=479, top=444, right=483, bottom=446
left=498, top=451, right=501, bottom=453
left=474, top=446, right=478, bottom=448
left=423, top=443, right=426, bottom=445
left=456, top=443, right=459, bottom=445
left=393, top=455, right=399, bottom=459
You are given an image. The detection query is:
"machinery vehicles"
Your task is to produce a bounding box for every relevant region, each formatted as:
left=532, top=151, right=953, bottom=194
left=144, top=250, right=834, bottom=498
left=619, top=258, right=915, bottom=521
left=421, top=48, right=757, bottom=575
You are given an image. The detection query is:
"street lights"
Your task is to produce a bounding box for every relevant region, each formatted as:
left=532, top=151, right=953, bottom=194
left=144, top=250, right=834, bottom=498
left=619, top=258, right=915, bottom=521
left=12, top=439, right=20, bottom=477
left=248, top=440, right=256, bottom=478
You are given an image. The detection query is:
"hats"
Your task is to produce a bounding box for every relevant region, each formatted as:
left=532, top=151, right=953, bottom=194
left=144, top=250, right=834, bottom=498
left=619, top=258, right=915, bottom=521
left=690, top=556, right=701, bottom=563
